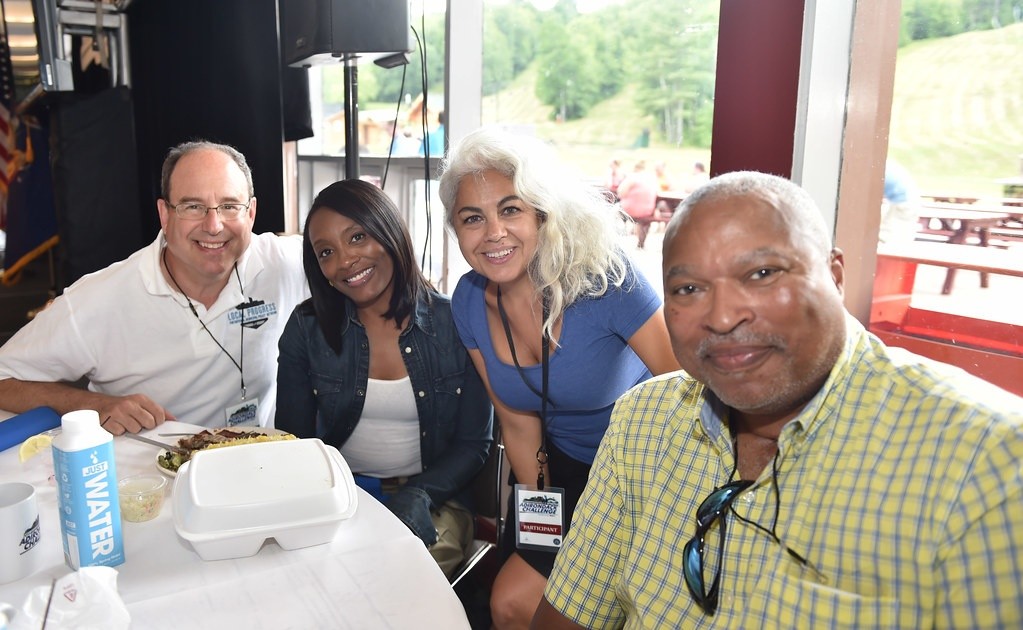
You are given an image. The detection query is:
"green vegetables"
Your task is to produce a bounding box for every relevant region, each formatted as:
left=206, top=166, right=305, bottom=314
left=157, top=452, right=187, bottom=472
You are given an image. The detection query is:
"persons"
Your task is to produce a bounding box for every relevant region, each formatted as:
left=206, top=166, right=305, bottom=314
left=275, top=179, right=495, bottom=579
left=606, top=158, right=704, bottom=219
left=528, top=171, right=1023, bottom=630
left=439, top=125, right=681, bottom=630
left=0, top=141, right=311, bottom=436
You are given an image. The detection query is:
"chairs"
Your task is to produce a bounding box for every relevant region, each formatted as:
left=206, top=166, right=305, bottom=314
left=428, top=408, right=504, bottom=630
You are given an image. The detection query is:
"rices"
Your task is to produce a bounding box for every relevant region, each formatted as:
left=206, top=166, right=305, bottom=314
left=120, top=483, right=162, bottom=522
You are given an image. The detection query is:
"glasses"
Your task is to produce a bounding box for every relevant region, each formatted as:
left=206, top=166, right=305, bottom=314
left=158, top=198, right=257, bottom=220
left=682, top=478, right=758, bottom=615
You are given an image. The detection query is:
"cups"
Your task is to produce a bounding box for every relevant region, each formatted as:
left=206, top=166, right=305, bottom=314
left=0, top=482, right=43, bottom=585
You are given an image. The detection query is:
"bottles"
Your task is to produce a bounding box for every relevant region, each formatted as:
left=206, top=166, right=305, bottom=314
left=51, top=409, right=126, bottom=573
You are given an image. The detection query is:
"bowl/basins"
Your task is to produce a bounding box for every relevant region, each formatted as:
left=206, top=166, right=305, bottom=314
left=116, top=473, right=167, bottom=523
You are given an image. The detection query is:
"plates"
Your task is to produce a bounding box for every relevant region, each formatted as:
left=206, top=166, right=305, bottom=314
left=155, top=428, right=297, bottom=477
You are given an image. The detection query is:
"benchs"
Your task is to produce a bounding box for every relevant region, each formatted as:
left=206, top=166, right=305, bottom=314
left=631, top=216, right=674, bottom=222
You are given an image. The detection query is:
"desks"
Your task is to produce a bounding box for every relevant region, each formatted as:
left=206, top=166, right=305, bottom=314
left=637, top=193, right=683, bottom=247
left=0, top=411, right=470, bottom=630
left=915, top=191, right=1023, bottom=293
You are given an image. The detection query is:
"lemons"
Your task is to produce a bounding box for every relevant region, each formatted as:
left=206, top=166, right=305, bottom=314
left=20, top=435, right=52, bottom=462
